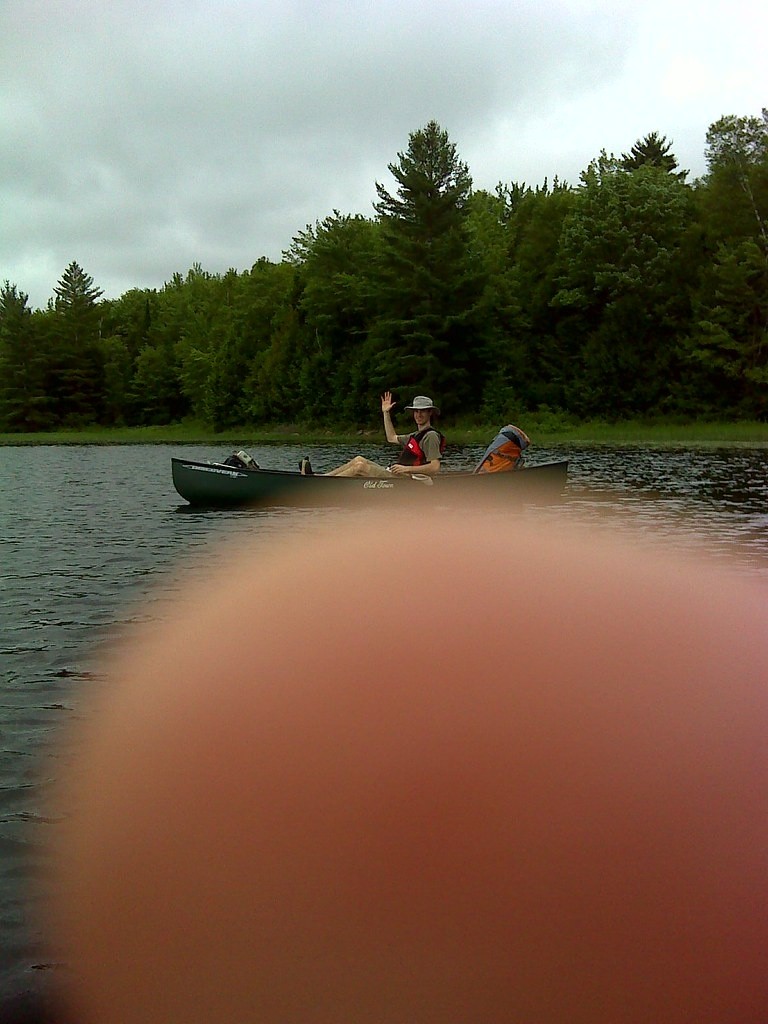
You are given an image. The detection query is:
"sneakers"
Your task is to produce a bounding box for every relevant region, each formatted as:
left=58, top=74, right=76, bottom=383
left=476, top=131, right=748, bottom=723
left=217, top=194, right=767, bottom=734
left=299, top=456, right=312, bottom=475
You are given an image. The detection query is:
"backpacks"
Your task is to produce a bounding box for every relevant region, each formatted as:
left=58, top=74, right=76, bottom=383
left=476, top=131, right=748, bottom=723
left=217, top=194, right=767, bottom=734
left=472, top=423, right=532, bottom=475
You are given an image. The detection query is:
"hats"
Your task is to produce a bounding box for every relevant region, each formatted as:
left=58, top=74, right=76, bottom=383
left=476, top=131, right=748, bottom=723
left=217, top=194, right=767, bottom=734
left=403, top=396, right=441, bottom=416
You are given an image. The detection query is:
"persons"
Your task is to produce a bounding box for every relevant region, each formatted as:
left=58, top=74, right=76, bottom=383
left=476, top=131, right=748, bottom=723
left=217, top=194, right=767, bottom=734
left=301, top=390, right=447, bottom=478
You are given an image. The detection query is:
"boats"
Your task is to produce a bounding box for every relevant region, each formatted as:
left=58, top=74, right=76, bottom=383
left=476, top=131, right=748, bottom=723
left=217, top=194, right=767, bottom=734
left=170, top=456, right=572, bottom=512
